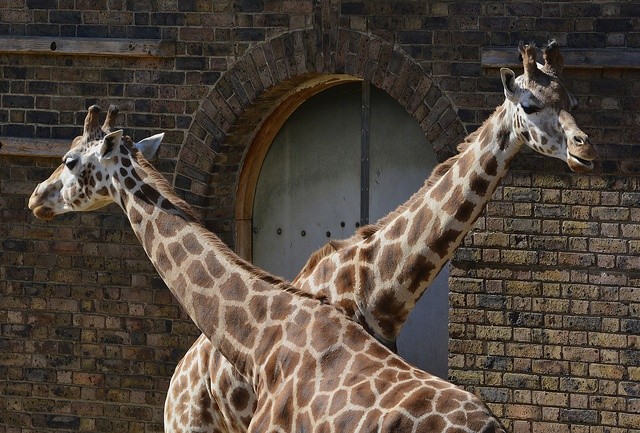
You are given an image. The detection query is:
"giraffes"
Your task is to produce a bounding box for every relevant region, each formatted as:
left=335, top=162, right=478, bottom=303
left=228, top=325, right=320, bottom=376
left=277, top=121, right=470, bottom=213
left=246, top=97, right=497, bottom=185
left=164, top=36, right=596, bottom=433
left=27, top=104, right=511, bottom=433
